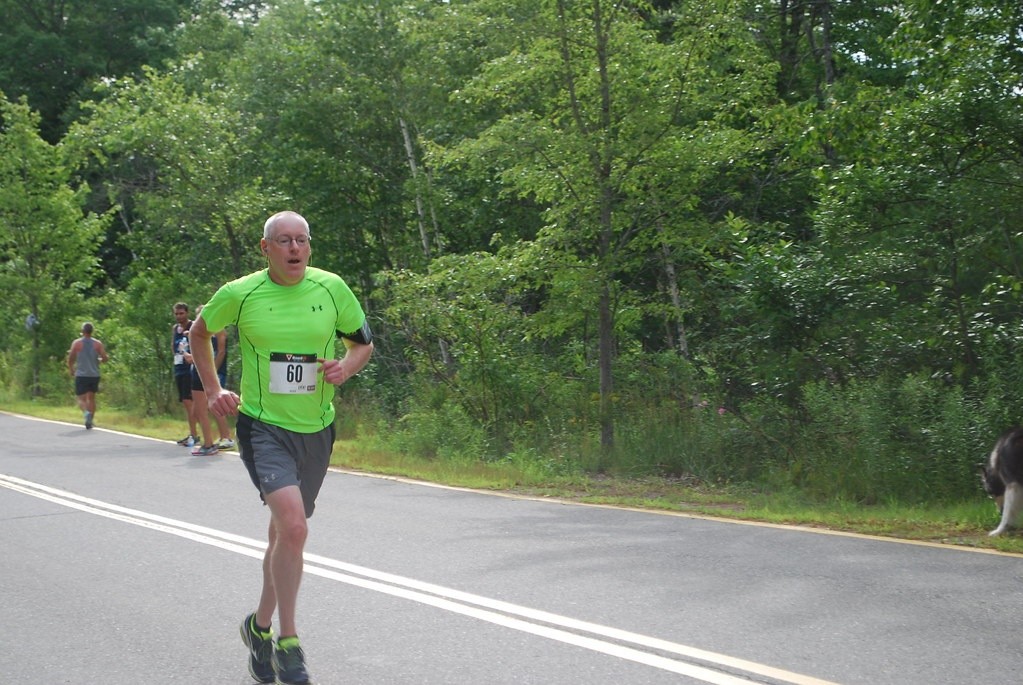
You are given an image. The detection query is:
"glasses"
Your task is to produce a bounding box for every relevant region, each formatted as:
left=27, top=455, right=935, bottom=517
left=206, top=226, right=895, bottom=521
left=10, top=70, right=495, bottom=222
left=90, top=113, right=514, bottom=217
left=265, top=234, right=311, bottom=247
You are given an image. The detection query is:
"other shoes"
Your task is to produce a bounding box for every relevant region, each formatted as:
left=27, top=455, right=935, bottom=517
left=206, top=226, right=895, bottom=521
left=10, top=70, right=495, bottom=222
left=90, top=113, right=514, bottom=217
left=85, top=412, right=93, bottom=429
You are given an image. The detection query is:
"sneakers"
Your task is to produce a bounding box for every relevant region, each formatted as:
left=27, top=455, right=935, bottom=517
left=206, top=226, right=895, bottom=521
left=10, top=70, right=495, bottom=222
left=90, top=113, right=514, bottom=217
left=191, top=444, right=218, bottom=455
left=177, top=437, right=188, bottom=444
left=239, top=612, right=275, bottom=683
left=273, top=636, right=311, bottom=685
left=182, top=436, right=200, bottom=447
left=214, top=438, right=235, bottom=451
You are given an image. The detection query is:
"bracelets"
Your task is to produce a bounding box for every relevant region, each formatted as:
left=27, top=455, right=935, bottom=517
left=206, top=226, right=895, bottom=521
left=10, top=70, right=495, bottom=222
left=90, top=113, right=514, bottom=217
left=180, top=349, right=186, bottom=355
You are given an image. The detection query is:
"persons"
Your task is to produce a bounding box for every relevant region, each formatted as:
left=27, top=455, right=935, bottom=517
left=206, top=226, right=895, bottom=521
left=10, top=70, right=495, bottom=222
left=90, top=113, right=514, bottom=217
left=188, top=211, right=373, bottom=685
left=69, top=323, right=108, bottom=429
left=170, top=302, right=237, bottom=457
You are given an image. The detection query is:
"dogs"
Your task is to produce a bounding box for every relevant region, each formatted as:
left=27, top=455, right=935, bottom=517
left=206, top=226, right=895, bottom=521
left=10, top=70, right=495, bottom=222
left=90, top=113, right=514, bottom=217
left=974, top=425, right=1023, bottom=536
